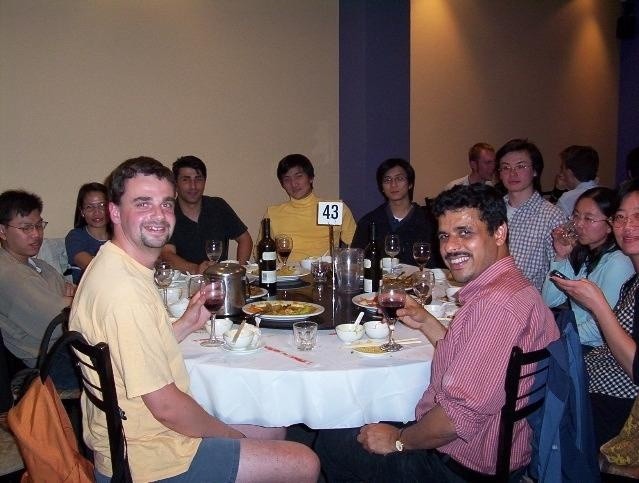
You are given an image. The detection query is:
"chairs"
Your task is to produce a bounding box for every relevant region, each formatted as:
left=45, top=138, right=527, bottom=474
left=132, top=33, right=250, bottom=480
left=498, top=318, right=581, bottom=483
left=0, top=268, right=132, bottom=483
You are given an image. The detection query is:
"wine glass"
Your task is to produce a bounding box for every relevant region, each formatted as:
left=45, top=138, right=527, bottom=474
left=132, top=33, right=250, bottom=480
left=153, top=235, right=467, bottom=356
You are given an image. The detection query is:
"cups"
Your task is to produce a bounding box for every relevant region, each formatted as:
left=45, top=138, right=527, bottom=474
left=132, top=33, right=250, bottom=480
left=557, top=220, right=583, bottom=247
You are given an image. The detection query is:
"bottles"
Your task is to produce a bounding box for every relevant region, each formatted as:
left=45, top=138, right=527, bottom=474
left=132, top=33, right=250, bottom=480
left=257, top=218, right=278, bottom=297
left=362, top=221, right=383, bottom=295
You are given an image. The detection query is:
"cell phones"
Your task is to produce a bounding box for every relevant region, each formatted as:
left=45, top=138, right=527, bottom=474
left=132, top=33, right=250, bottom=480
left=549, top=270, right=570, bottom=280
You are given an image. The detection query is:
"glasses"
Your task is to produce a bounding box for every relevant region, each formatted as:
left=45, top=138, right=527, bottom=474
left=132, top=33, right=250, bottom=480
left=495, top=159, right=533, bottom=175
left=380, top=173, right=407, bottom=185
left=78, top=202, right=108, bottom=214
left=609, top=212, right=639, bottom=231
left=3, top=219, right=49, bottom=231
left=567, top=208, right=609, bottom=228
